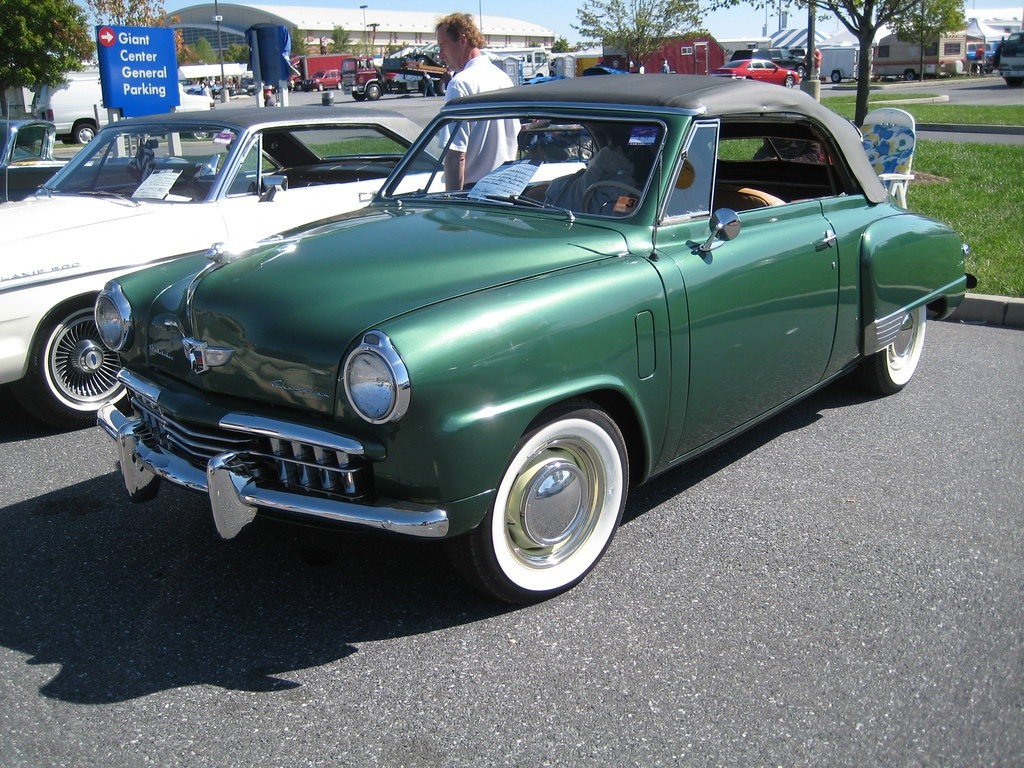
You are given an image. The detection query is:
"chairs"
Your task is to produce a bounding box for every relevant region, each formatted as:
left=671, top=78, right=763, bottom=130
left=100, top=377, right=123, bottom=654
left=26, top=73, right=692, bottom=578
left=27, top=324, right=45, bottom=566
left=858, top=107, right=916, bottom=211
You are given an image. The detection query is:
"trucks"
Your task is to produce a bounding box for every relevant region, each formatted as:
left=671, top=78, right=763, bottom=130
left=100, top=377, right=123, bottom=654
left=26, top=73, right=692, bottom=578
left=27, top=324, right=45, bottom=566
left=339, top=57, right=451, bottom=102
left=288, top=54, right=353, bottom=91
left=998, top=31, right=1024, bottom=86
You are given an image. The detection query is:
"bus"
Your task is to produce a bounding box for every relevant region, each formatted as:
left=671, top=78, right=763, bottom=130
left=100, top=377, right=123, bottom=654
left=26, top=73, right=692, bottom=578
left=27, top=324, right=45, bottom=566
left=486, top=47, right=549, bottom=79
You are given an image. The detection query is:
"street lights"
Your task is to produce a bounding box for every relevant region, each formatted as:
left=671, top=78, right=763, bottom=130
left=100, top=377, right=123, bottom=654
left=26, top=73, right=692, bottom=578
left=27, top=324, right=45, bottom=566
left=367, top=23, right=379, bottom=47
left=360, top=5, right=369, bottom=57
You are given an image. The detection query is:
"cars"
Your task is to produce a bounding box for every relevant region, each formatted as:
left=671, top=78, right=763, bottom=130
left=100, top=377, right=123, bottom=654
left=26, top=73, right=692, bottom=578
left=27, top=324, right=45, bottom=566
left=93, top=73, right=978, bottom=603
left=1, top=103, right=587, bottom=418
left=408, top=42, right=446, bottom=68
left=549, top=58, right=558, bottom=77
left=714, top=59, right=800, bottom=88
left=186, top=83, right=236, bottom=98
left=0, top=119, right=193, bottom=204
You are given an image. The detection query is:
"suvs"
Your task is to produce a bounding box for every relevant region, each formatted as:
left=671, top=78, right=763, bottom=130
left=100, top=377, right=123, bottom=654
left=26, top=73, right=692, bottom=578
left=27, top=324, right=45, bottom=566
left=731, top=50, right=806, bottom=77
left=301, top=69, right=343, bottom=91
left=966, top=42, right=997, bottom=74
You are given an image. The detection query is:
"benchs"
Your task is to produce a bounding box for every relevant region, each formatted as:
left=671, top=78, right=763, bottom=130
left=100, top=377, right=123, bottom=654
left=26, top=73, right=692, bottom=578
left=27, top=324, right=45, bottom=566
left=529, top=180, right=788, bottom=211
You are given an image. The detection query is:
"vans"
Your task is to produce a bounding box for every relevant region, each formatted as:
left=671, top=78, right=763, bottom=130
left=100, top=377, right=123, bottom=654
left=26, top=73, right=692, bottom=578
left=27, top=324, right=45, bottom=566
left=37, top=65, right=214, bottom=147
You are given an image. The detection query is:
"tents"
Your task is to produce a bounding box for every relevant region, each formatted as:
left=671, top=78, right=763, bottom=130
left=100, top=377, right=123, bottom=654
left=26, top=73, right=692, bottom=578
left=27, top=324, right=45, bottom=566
left=966, top=19, right=1010, bottom=63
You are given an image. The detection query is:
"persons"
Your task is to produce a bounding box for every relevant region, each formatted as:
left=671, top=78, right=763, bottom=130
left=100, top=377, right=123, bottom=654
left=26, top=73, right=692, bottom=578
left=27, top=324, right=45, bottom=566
left=518, top=119, right=591, bottom=161
left=753, top=138, right=821, bottom=163
left=814, top=48, right=822, bottom=80
left=264, top=89, right=274, bottom=107
left=190, top=78, right=212, bottom=98
left=975, top=47, right=984, bottom=59
left=444, top=66, right=454, bottom=89
left=422, top=75, right=436, bottom=97
left=662, top=60, right=669, bottom=74
left=434, top=13, right=522, bottom=192
left=290, top=79, right=294, bottom=90
